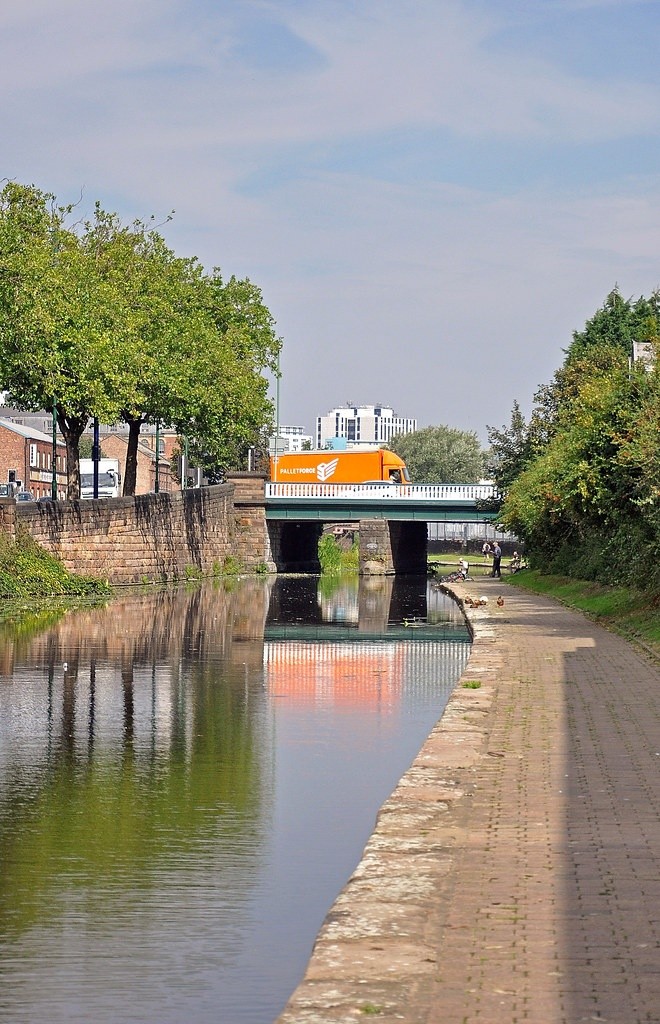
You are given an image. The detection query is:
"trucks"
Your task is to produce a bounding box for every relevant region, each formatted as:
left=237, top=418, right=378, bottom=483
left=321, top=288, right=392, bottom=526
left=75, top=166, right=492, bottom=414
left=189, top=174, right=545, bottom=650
left=269, top=448, right=413, bottom=484
left=79, top=458, right=122, bottom=500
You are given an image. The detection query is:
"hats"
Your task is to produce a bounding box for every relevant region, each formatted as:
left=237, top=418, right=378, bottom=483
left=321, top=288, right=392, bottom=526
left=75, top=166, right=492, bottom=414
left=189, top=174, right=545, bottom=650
left=492, top=541, right=498, bottom=545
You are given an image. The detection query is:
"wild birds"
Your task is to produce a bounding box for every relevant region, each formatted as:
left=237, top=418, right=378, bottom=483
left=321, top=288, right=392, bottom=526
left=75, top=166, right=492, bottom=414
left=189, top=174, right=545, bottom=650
left=465, top=596, right=487, bottom=609
left=497, top=595, right=504, bottom=607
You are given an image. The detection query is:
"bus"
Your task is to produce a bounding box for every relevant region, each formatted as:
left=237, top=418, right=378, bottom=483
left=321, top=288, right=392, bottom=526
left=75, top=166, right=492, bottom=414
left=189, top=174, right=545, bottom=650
left=0, top=483, right=14, bottom=497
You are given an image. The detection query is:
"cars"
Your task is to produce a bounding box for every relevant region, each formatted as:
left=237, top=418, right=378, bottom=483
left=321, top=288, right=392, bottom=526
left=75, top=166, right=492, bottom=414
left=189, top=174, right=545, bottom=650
left=14, top=492, right=35, bottom=503
left=36, top=496, right=59, bottom=502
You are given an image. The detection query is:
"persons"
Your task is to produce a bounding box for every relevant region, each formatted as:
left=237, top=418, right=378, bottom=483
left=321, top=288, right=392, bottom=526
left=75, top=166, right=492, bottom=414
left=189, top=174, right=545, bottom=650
left=512, top=552, right=520, bottom=559
left=489, top=540, right=502, bottom=578
left=390, top=471, right=399, bottom=481
left=458, top=558, right=468, bottom=574
left=483, top=541, right=491, bottom=559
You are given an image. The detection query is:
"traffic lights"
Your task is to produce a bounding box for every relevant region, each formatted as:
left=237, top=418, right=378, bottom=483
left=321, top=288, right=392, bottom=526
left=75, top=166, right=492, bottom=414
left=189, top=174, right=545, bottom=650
left=8, top=469, right=16, bottom=483
